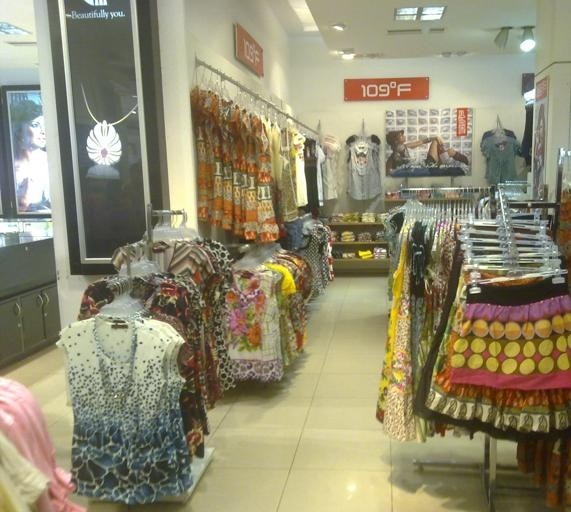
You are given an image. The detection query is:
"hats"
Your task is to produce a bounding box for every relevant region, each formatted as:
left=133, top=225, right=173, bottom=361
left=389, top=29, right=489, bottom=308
left=387, top=130, right=403, bottom=145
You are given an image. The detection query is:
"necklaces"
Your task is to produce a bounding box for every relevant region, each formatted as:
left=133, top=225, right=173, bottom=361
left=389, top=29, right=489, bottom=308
left=79, top=81, right=137, bottom=167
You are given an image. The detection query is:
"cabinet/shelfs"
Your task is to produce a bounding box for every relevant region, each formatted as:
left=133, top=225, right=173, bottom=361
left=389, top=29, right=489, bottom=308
left=327, top=222, right=390, bottom=273
left=0, top=239, right=60, bottom=370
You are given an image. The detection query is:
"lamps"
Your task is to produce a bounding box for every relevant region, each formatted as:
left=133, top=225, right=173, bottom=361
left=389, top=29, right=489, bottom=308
left=494, top=26, right=536, bottom=53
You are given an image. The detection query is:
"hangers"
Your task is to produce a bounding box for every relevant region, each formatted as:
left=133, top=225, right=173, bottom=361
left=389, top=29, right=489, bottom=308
left=193, top=58, right=320, bottom=147
left=95, top=208, right=282, bottom=324
left=399, top=180, right=568, bottom=296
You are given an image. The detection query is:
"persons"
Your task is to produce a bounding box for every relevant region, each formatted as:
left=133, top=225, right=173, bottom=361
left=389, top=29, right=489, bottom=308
left=9, top=98, right=54, bottom=214
left=386, top=129, right=470, bottom=177
